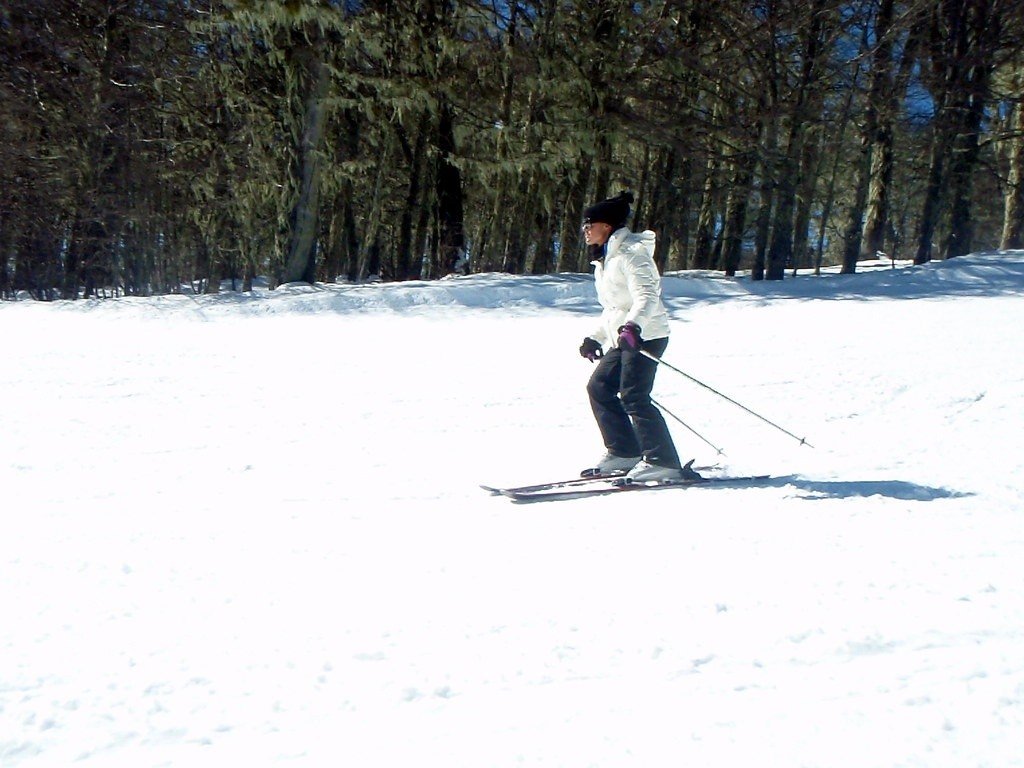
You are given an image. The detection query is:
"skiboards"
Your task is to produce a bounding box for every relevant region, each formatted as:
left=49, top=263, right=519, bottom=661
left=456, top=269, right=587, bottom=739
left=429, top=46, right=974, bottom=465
left=476, top=461, right=773, bottom=502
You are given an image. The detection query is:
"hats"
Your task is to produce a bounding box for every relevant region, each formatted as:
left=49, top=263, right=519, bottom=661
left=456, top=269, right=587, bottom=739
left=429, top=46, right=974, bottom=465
left=583, top=190, right=634, bottom=227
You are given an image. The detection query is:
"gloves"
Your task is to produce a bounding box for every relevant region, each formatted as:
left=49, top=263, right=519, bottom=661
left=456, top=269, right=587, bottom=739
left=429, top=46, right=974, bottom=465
left=580, top=337, right=603, bottom=363
left=617, top=321, right=644, bottom=353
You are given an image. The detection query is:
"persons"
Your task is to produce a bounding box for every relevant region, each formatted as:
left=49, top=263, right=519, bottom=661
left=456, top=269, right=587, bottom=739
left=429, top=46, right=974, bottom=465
left=579, top=191, right=682, bottom=478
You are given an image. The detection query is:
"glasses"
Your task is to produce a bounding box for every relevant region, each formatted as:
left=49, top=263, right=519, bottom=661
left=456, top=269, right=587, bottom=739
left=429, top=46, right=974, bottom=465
left=582, top=218, right=591, bottom=231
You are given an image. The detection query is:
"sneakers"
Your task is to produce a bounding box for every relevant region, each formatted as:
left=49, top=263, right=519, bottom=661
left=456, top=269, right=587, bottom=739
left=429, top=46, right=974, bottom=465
left=626, top=461, right=683, bottom=481
left=595, top=453, right=642, bottom=473
